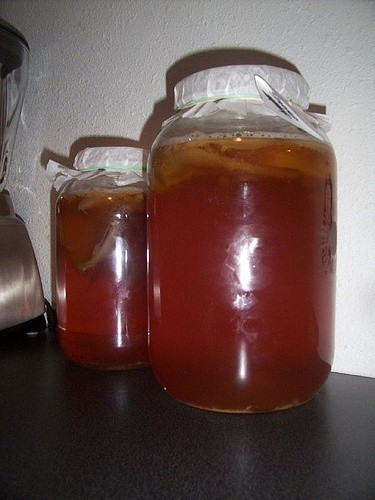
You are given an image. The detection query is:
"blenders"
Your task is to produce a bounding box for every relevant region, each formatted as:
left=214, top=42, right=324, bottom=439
left=0, top=16, right=50, bottom=343
